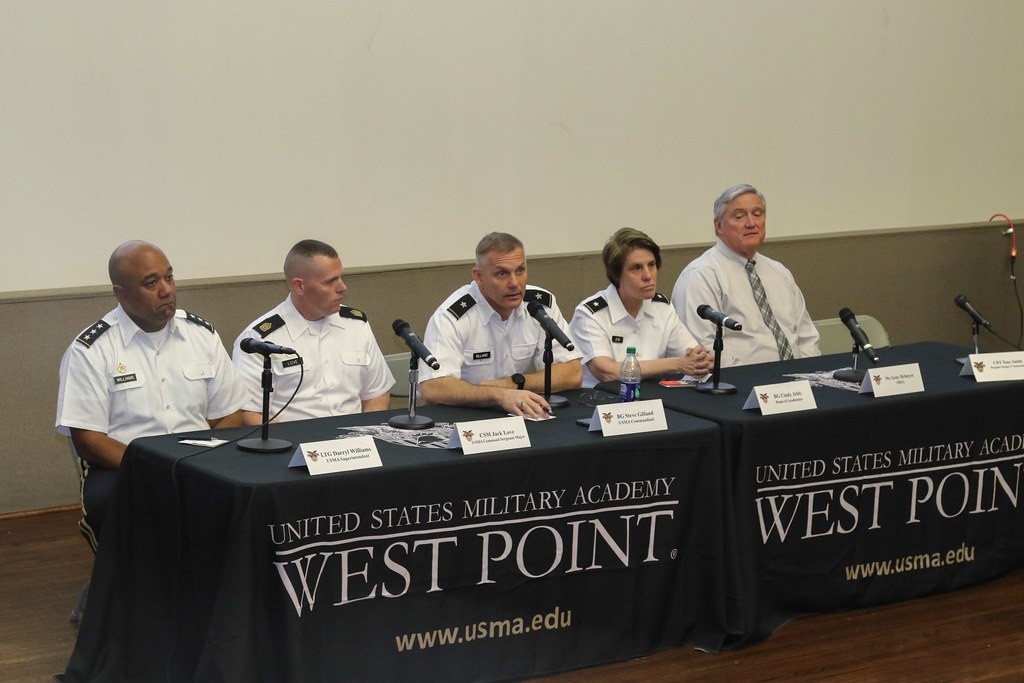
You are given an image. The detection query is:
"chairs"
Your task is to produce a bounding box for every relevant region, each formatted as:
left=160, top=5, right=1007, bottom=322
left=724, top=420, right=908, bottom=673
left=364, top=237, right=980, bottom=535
left=385, top=315, right=892, bottom=396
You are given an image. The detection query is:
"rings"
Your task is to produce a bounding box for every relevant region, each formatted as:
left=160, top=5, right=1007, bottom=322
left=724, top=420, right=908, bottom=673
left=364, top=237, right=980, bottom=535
left=520, top=401, right=525, bottom=408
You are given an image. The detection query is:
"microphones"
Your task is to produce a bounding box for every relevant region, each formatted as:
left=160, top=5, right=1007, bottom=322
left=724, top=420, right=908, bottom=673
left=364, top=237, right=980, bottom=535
left=840, top=307, right=879, bottom=364
left=527, top=301, right=575, bottom=351
left=697, top=304, right=742, bottom=331
left=392, top=319, right=440, bottom=370
left=955, top=295, right=991, bottom=328
left=240, top=338, right=296, bottom=356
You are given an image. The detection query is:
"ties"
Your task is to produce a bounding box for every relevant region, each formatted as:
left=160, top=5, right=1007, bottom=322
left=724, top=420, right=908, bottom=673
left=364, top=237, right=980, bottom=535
left=745, top=261, right=795, bottom=361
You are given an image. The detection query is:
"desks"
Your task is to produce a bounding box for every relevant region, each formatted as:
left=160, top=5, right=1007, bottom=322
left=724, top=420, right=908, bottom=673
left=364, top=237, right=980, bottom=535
left=56, top=388, right=729, bottom=683
left=596, top=339, right=1024, bottom=653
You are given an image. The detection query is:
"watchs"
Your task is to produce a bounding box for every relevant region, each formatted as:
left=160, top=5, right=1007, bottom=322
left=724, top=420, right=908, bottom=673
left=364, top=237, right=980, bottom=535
left=511, top=373, right=526, bottom=390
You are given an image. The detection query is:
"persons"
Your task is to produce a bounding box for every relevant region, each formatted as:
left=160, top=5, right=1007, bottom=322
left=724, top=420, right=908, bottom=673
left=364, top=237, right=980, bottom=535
left=668, top=182, right=823, bottom=368
left=50, top=239, right=255, bottom=556
left=417, top=232, right=584, bottom=419
left=568, top=226, right=713, bottom=389
left=232, top=239, right=397, bottom=426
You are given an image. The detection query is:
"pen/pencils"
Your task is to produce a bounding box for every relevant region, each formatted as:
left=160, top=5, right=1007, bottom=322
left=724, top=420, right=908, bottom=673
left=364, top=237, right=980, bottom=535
left=539, top=404, right=553, bottom=415
left=177, top=435, right=218, bottom=441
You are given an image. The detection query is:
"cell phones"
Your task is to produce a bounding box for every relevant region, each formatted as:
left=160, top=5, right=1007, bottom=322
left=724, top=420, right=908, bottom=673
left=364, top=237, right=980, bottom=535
left=659, top=380, right=695, bottom=388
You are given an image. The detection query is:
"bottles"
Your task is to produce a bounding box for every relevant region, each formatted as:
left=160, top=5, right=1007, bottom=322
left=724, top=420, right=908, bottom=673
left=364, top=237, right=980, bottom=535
left=620, top=347, right=642, bottom=403
